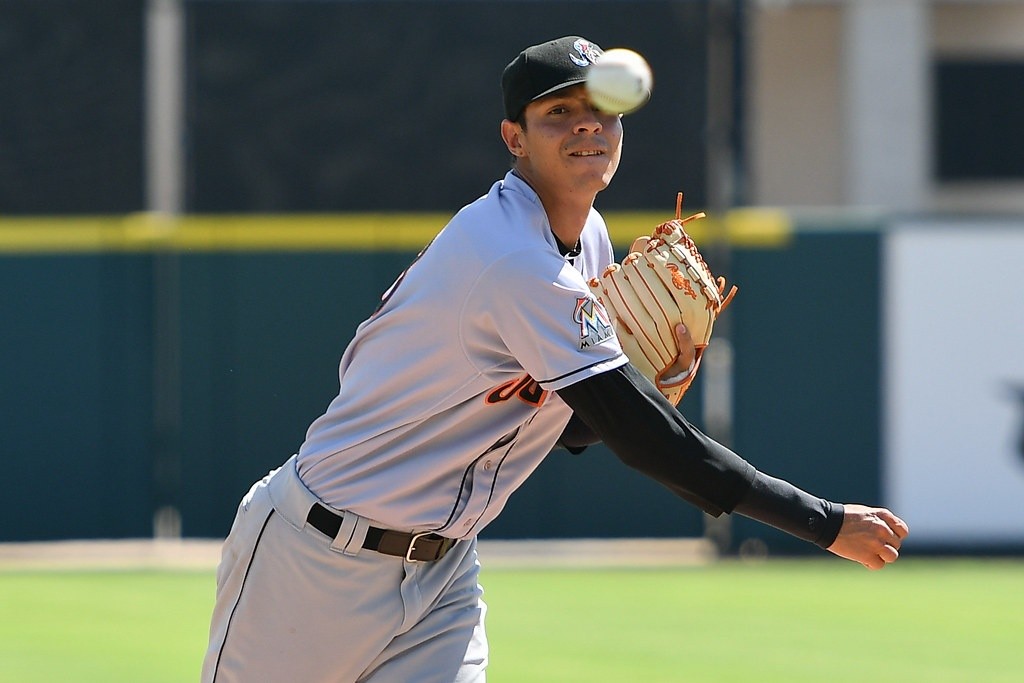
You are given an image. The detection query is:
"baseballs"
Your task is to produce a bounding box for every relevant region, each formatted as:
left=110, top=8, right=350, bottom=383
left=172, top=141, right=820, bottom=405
left=585, top=45, right=657, bottom=117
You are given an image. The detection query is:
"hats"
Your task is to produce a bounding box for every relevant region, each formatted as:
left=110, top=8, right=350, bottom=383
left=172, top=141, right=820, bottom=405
left=501, top=36, right=651, bottom=122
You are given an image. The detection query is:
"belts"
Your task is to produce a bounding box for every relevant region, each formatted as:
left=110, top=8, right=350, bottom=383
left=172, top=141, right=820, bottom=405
left=307, top=503, right=454, bottom=562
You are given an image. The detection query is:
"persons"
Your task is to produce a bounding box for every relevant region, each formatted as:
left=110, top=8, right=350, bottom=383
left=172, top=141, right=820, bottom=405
left=199, top=36, right=909, bottom=682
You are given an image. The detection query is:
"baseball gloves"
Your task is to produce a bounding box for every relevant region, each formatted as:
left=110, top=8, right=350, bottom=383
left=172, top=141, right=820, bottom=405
left=585, top=188, right=740, bottom=407
